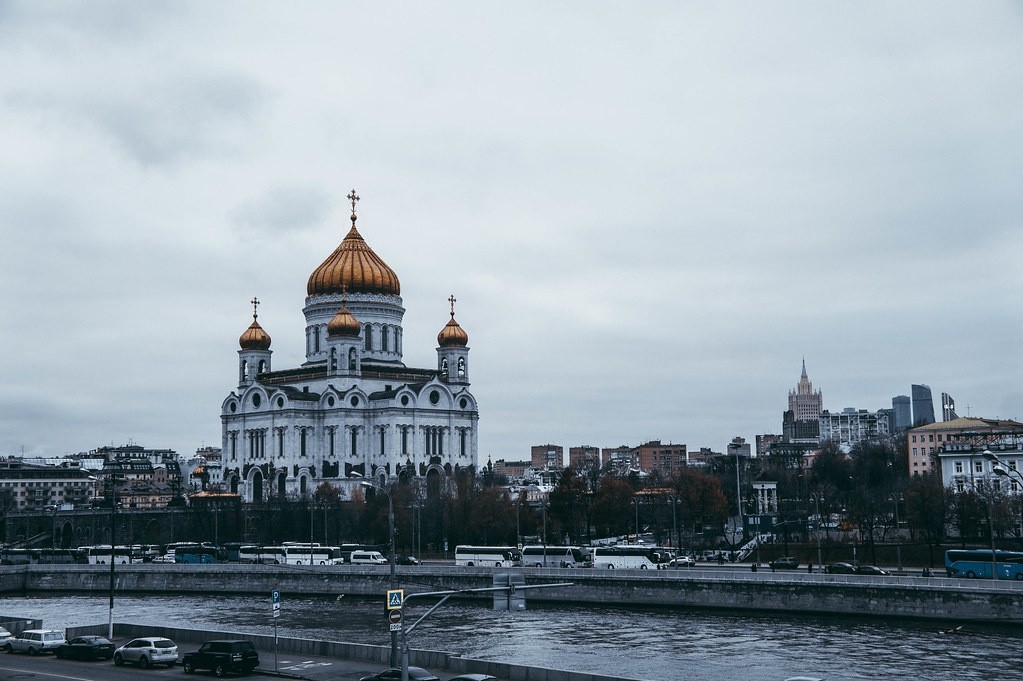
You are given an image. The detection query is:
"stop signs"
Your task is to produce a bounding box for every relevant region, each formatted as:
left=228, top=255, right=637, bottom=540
left=389, top=610, right=401, bottom=623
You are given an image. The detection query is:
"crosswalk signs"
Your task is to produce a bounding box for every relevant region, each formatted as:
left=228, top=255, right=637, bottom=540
left=387, top=591, right=403, bottom=611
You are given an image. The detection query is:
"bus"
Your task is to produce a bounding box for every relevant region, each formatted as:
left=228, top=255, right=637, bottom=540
left=522, top=545, right=593, bottom=568
left=593, top=546, right=672, bottom=570
left=0, top=542, right=390, bottom=566
left=455, top=544, right=522, bottom=567
left=945, top=548, right=1023, bottom=581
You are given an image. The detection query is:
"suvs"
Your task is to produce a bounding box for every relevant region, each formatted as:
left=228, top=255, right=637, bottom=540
left=8, top=630, right=65, bottom=656
left=670, top=556, right=695, bottom=568
left=396, top=556, right=424, bottom=566
left=178, top=640, right=260, bottom=677
left=769, top=557, right=800, bottom=570
left=114, top=635, right=179, bottom=669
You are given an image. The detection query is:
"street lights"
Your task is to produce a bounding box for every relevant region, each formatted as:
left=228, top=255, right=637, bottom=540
left=308, top=503, right=333, bottom=549
left=212, top=505, right=222, bottom=565
left=351, top=472, right=397, bottom=667
left=511, top=497, right=552, bottom=568
left=78, top=468, right=115, bottom=639
left=242, top=504, right=251, bottom=542
left=665, top=494, right=682, bottom=548
left=631, top=496, right=643, bottom=544
left=809, top=491, right=826, bottom=566
left=409, top=502, right=424, bottom=567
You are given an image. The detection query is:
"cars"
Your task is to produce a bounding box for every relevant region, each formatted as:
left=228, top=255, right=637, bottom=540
left=55, top=635, right=116, bottom=661
left=0, top=626, right=14, bottom=649
left=825, top=563, right=891, bottom=575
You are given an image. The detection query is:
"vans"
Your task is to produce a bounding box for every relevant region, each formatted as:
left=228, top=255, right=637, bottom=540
left=350, top=550, right=388, bottom=566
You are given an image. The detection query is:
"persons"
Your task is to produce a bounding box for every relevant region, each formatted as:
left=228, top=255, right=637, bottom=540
left=808, top=560, right=812, bottom=573
left=923, top=568, right=953, bottom=577
left=657, top=562, right=667, bottom=570
left=717, top=552, right=757, bottom=571
